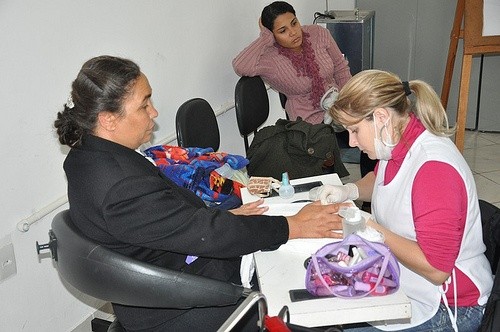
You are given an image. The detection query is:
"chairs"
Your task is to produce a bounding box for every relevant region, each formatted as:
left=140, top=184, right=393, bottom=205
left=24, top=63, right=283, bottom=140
left=176, top=98, right=220, bottom=152
left=234, top=72, right=269, bottom=153
left=34, top=209, right=268, bottom=332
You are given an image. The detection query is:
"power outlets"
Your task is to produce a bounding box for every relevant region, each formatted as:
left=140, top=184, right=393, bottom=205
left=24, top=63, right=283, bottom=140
left=0, top=244, right=17, bottom=281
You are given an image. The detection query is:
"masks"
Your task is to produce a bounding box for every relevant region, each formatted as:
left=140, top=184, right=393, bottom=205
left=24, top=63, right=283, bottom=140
left=373, top=113, right=392, bottom=161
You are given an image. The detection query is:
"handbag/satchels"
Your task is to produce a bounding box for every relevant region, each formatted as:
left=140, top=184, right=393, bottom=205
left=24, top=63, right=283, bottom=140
left=304, top=232, right=400, bottom=298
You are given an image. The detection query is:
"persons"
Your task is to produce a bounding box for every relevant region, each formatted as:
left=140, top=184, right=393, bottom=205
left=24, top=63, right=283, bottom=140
left=232, top=1, right=353, bottom=125
left=55, top=56, right=352, bottom=332
left=329, top=69, right=495, bottom=332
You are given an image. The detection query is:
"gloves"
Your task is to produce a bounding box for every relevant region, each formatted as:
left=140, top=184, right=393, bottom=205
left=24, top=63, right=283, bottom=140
left=338, top=208, right=375, bottom=227
left=315, top=183, right=359, bottom=206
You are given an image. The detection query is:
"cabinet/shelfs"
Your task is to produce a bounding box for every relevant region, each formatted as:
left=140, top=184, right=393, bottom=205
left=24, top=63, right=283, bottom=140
left=315, top=9, right=377, bottom=76
